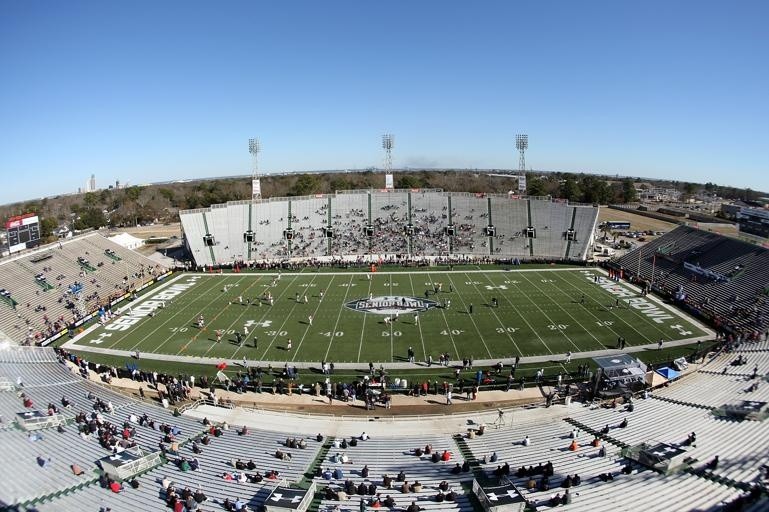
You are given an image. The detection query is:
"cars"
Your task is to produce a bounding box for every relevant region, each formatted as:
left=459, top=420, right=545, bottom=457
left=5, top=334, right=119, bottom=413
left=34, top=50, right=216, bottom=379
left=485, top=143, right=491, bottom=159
left=618, top=230, right=666, bottom=238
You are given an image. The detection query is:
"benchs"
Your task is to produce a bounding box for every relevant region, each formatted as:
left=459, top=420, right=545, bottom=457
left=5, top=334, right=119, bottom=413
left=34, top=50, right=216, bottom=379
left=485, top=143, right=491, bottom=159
left=609, top=224, right=769, bottom=341
left=0, top=231, right=172, bottom=346
left=0, top=340, right=769, bottom=511
left=175, top=193, right=600, bottom=268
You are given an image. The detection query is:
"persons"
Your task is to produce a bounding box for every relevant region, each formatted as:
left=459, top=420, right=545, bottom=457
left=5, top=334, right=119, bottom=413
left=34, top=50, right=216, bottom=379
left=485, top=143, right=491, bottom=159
left=181, top=200, right=594, bottom=269
left=577, top=227, right=769, bottom=418
left=11, top=237, right=181, bottom=346
left=13, top=343, right=769, bottom=511
left=191, top=275, right=499, bottom=369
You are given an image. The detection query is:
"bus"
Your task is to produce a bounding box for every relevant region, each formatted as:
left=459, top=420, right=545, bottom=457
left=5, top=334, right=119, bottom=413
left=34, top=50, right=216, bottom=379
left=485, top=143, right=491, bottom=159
left=607, top=221, right=630, bottom=230
